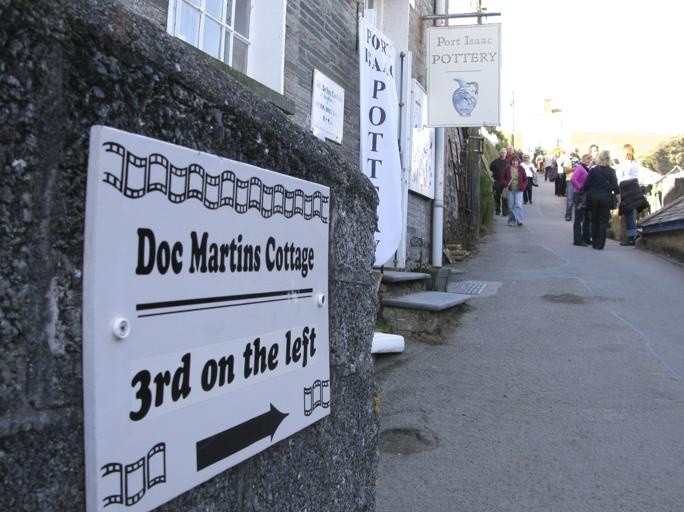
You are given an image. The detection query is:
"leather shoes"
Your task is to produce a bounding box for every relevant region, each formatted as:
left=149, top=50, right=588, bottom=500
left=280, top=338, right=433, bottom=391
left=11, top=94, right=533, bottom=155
left=518, top=223, right=522, bottom=225
left=573, top=240, right=604, bottom=250
left=619, top=239, right=635, bottom=246
left=565, top=216, right=571, bottom=221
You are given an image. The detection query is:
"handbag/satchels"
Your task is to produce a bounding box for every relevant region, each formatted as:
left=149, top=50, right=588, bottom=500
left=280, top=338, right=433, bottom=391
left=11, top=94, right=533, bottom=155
left=502, top=187, right=508, bottom=198
left=532, top=178, right=539, bottom=187
left=611, top=194, right=617, bottom=210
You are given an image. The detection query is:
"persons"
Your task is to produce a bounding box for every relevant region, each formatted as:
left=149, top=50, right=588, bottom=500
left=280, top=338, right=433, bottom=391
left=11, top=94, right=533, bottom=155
left=488, top=142, right=651, bottom=252
